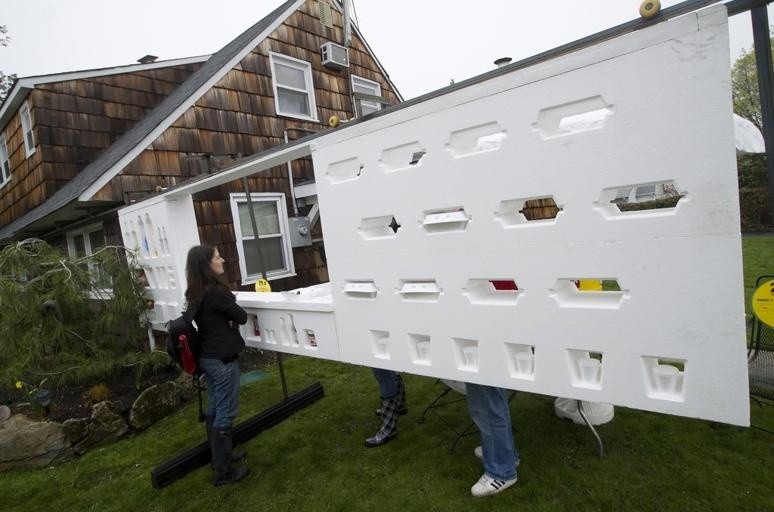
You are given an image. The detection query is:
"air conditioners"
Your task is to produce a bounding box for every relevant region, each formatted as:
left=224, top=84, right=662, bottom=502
left=320, top=40, right=351, bottom=71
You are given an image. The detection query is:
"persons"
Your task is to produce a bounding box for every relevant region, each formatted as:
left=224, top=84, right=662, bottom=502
left=463, top=381, right=521, bottom=498
left=183, top=242, right=252, bottom=488
left=362, top=367, right=408, bottom=449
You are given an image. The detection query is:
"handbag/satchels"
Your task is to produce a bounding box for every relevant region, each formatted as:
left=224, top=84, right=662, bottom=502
left=164, top=306, right=206, bottom=423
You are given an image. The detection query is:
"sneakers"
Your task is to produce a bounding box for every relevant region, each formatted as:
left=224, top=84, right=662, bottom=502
left=471, top=474, right=517, bottom=497
left=474, top=446, right=519, bottom=468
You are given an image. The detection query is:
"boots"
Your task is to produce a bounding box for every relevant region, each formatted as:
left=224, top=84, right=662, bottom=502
left=365, top=397, right=398, bottom=447
left=211, top=426, right=249, bottom=486
left=376, top=375, right=407, bottom=416
left=206, top=415, right=247, bottom=471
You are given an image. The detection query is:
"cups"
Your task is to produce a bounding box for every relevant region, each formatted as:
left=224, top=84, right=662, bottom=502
left=376, top=337, right=389, bottom=358
left=463, top=345, right=477, bottom=367
left=417, top=341, right=430, bottom=361
left=514, top=352, right=534, bottom=376
left=577, top=358, right=601, bottom=385
left=652, top=364, right=679, bottom=394
